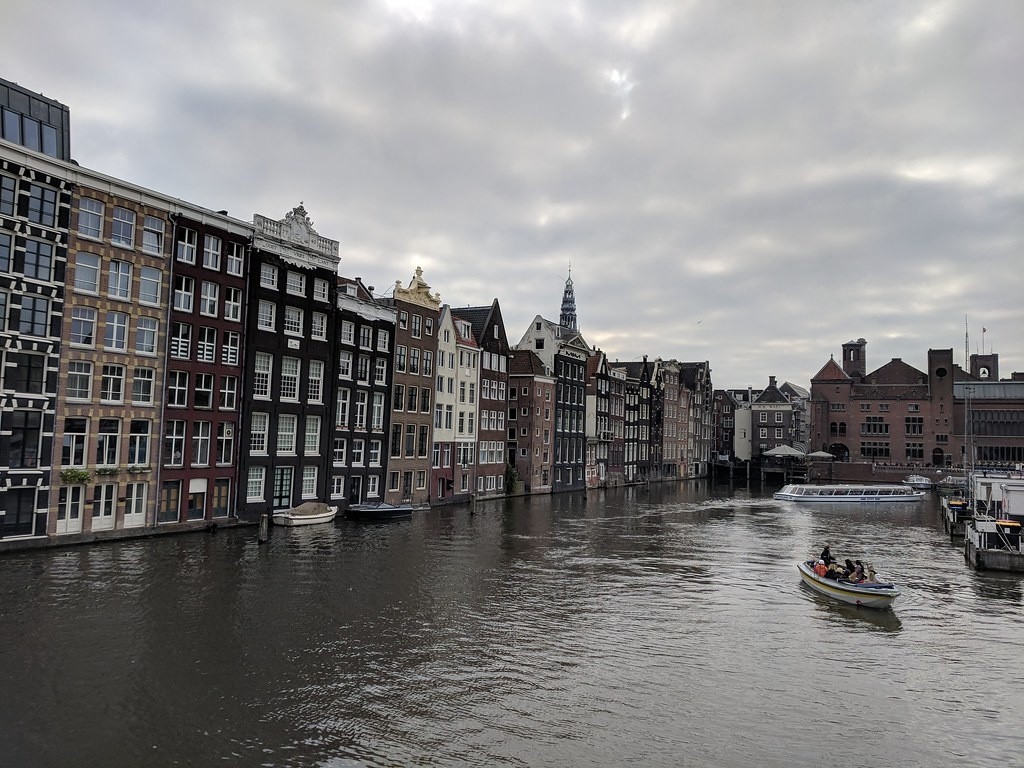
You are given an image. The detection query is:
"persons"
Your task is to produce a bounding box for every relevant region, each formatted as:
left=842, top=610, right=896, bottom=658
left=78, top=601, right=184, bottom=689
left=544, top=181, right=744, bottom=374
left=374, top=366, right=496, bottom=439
left=814, top=545, right=862, bottom=583
left=884, top=461, right=931, bottom=470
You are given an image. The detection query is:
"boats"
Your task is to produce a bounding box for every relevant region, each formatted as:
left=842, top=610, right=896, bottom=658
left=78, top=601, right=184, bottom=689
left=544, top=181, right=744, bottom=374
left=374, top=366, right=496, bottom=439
left=272, top=503, right=338, bottom=526
left=964, top=513, right=1024, bottom=573
left=902, top=475, right=934, bottom=489
left=773, top=483, right=926, bottom=502
left=940, top=495, right=979, bottom=538
left=344, top=503, right=413, bottom=522
left=798, top=559, right=901, bottom=609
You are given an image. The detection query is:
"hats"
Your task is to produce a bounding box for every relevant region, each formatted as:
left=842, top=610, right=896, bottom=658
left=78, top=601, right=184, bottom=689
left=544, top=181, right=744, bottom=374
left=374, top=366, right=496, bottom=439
left=819, top=560, right=825, bottom=564
